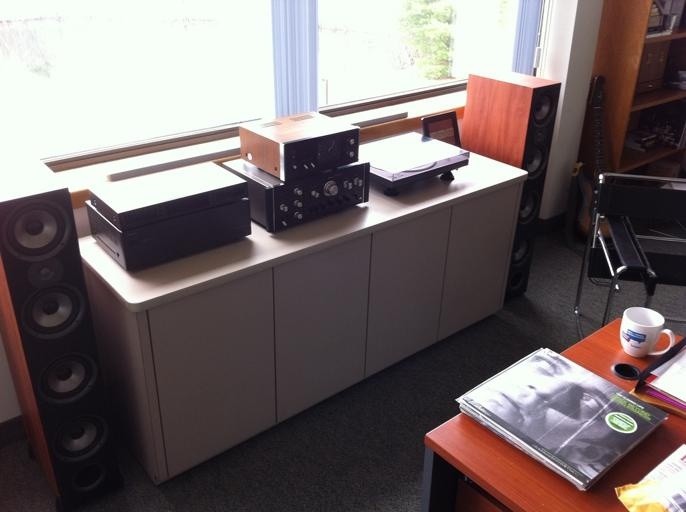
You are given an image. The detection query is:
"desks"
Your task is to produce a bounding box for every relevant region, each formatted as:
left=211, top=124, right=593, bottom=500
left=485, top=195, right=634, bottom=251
left=419, top=317, right=685, bottom=512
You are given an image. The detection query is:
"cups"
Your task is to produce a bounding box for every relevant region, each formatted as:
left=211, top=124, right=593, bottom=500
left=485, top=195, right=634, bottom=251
left=620, top=306, right=676, bottom=358
left=665, top=12, right=680, bottom=35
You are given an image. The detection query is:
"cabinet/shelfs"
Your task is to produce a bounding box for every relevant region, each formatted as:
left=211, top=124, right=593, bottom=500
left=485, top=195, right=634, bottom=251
left=273, top=203, right=369, bottom=421
left=565, top=0, right=686, bottom=178
left=437, top=151, right=528, bottom=345
left=79, top=219, right=275, bottom=488
left=363, top=173, right=452, bottom=380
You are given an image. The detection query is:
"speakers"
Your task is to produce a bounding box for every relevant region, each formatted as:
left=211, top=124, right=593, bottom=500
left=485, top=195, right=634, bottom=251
left=0, top=152, right=128, bottom=510
left=462, top=68, right=561, bottom=303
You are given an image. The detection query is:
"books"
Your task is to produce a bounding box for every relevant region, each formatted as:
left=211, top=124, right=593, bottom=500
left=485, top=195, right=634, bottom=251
left=632, top=338, right=686, bottom=417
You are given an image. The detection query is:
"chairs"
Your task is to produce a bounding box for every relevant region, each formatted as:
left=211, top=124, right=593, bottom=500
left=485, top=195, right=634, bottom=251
left=574, top=172, right=685, bottom=342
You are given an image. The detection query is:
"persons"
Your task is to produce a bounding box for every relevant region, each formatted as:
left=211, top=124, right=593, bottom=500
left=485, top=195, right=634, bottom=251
left=467, top=347, right=650, bottom=465
